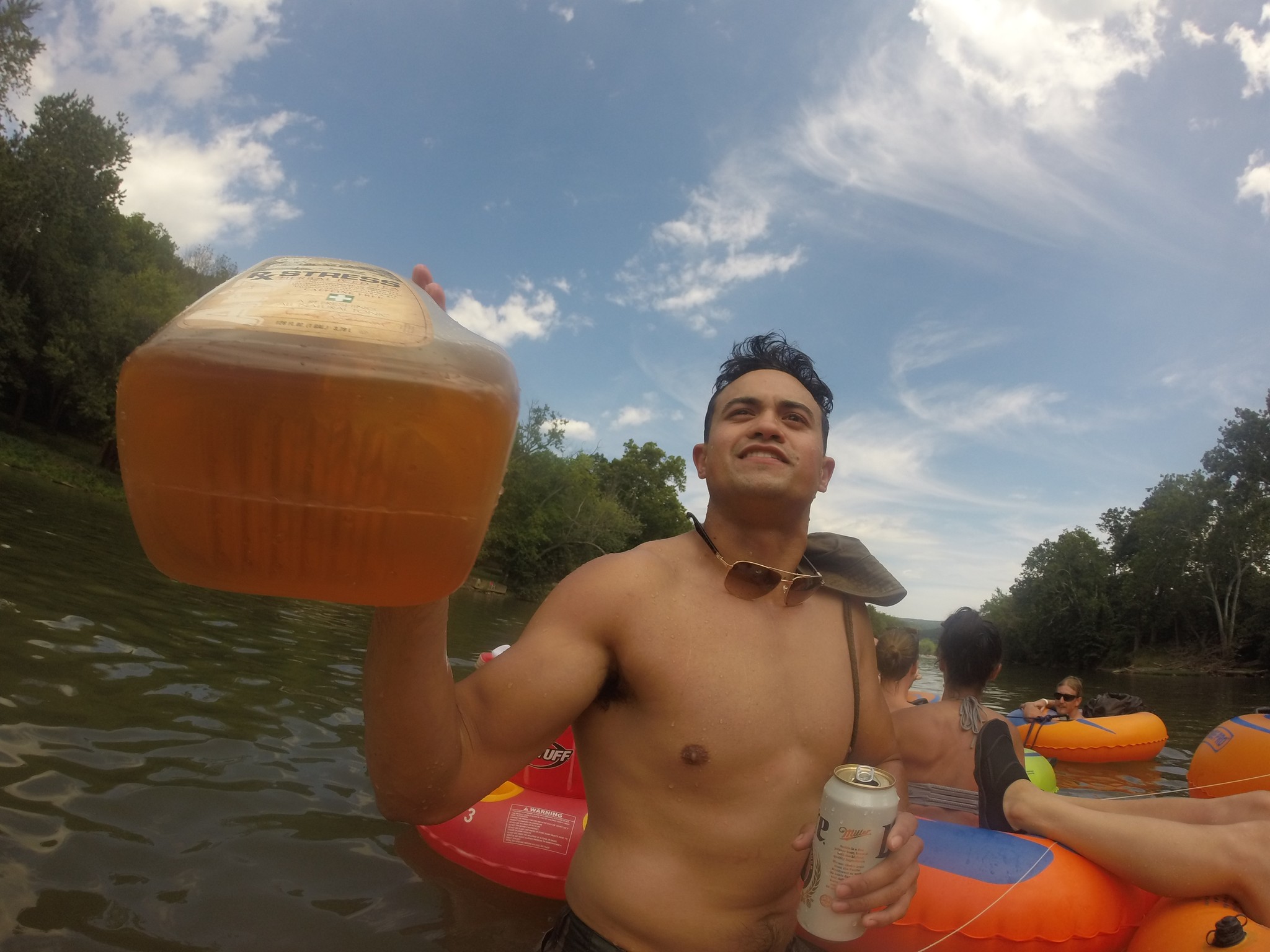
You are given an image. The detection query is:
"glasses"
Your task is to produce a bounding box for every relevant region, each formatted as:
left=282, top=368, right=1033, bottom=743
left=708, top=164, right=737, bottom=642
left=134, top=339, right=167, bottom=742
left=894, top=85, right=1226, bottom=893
left=880, top=627, right=920, bottom=645
left=686, top=510, right=824, bottom=605
left=1053, top=692, right=1079, bottom=702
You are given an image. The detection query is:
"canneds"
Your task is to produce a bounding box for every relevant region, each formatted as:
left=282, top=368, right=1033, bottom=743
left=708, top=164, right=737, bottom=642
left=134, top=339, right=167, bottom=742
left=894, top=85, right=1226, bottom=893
left=796, top=764, right=899, bottom=941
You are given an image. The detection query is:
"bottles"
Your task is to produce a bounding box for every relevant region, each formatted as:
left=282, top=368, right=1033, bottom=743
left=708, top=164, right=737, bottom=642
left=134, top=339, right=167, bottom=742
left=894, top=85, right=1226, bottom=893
left=113, top=258, right=520, bottom=605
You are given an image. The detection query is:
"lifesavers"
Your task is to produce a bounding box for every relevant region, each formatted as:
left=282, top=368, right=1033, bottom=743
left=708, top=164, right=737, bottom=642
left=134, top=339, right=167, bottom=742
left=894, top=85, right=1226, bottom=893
left=1024, top=748, right=1059, bottom=793
left=1127, top=894, right=1270, bottom=952
left=416, top=724, right=587, bottom=901
left=802, top=803, right=1159, bottom=952
left=1187, top=714, right=1270, bottom=797
left=1007, top=709, right=1169, bottom=761
left=907, top=691, right=943, bottom=706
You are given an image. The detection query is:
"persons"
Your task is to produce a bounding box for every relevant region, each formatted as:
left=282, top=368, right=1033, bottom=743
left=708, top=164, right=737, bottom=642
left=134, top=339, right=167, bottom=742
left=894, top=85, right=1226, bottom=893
left=1020, top=676, right=1085, bottom=723
left=874, top=627, right=923, bottom=713
left=974, top=719, right=1270, bottom=928
left=362, top=261, right=924, bottom=952
left=888, top=605, right=1025, bottom=830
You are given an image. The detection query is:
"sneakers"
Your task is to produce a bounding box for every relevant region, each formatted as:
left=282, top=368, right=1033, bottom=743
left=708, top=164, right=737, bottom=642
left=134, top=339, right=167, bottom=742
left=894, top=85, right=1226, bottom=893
left=975, top=719, right=1031, bottom=833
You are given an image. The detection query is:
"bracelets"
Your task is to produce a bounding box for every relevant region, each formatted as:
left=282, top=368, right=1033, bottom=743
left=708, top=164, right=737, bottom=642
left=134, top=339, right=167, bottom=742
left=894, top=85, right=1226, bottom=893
left=1042, top=698, right=1048, bottom=706
